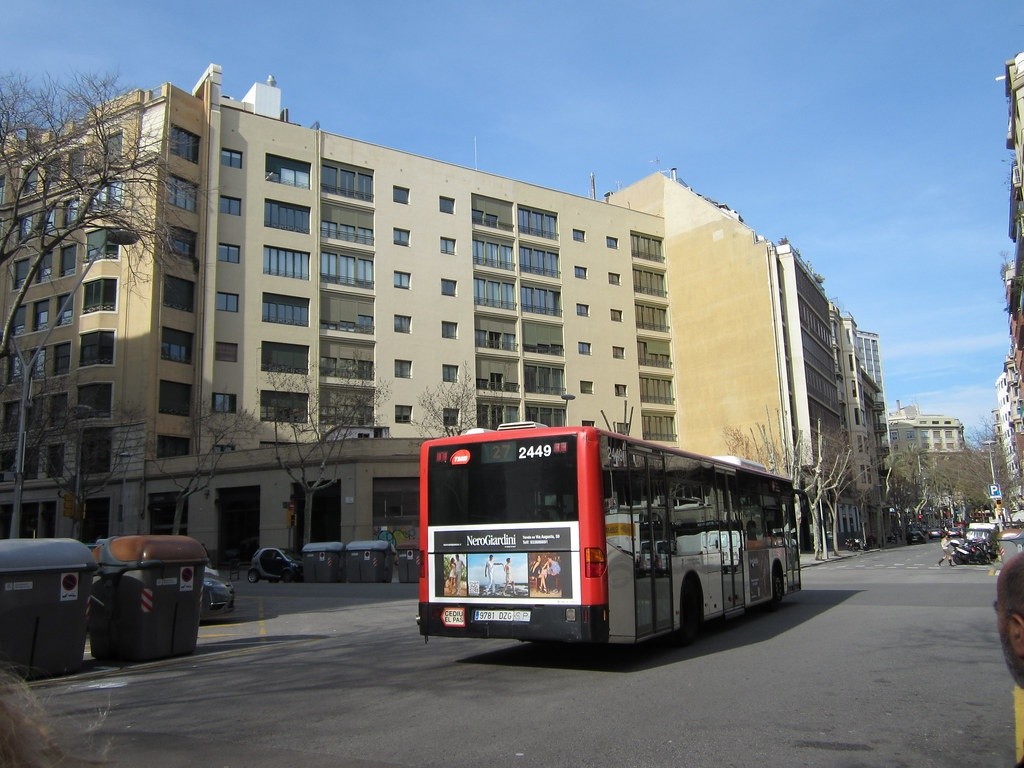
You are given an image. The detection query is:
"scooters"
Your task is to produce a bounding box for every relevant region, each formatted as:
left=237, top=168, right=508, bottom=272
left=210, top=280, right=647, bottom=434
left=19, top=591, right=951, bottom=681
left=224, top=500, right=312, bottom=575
left=949, top=536, right=993, bottom=566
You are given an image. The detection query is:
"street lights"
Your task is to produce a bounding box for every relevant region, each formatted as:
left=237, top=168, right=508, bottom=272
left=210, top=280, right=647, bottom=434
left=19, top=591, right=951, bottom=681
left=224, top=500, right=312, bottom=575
left=559, top=394, right=577, bottom=427
left=1, top=227, right=141, bottom=537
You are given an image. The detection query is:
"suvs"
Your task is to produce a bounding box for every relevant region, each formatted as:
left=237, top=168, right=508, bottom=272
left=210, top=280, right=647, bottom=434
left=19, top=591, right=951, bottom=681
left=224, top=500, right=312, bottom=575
left=906, top=530, right=927, bottom=546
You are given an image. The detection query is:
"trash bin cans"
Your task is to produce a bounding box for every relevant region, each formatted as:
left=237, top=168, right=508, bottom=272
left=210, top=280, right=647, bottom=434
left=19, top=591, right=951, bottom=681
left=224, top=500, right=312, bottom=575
left=301, top=542, right=347, bottom=583
left=88, top=535, right=211, bottom=667
left=395, top=540, right=420, bottom=583
left=996, top=529, right=1024, bottom=566
left=344, top=540, right=397, bottom=583
left=0, top=538, right=100, bottom=681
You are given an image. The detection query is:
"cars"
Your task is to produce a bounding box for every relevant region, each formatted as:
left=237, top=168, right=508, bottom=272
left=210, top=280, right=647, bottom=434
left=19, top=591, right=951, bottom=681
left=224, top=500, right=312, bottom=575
left=84, top=542, right=237, bottom=625
left=248, top=547, right=303, bottom=583
left=929, top=528, right=944, bottom=540
left=947, top=525, right=964, bottom=538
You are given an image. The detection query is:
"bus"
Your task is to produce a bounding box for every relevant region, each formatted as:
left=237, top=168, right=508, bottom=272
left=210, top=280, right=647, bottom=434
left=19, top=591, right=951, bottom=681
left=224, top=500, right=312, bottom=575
left=415, top=419, right=801, bottom=648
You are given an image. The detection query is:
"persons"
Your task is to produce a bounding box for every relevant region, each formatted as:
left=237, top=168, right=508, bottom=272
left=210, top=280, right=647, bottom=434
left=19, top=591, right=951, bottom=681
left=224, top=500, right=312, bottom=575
left=448, top=554, right=465, bottom=595
left=484, top=555, right=518, bottom=596
left=532, top=555, right=560, bottom=593
left=994, top=552, right=1024, bottom=768
left=938, top=532, right=955, bottom=566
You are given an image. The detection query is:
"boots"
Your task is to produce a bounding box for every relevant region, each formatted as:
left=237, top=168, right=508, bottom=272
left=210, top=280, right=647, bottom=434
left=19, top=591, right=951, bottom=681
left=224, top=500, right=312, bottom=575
left=938, top=558, right=944, bottom=566
left=948, top=560, right=955, bottom=567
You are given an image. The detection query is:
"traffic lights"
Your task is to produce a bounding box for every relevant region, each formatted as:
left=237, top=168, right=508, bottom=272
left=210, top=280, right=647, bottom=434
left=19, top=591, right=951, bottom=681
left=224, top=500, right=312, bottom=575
left=997, top=499, right=1001, bottom=505
left=918, top=515, right=923, bottom=519
